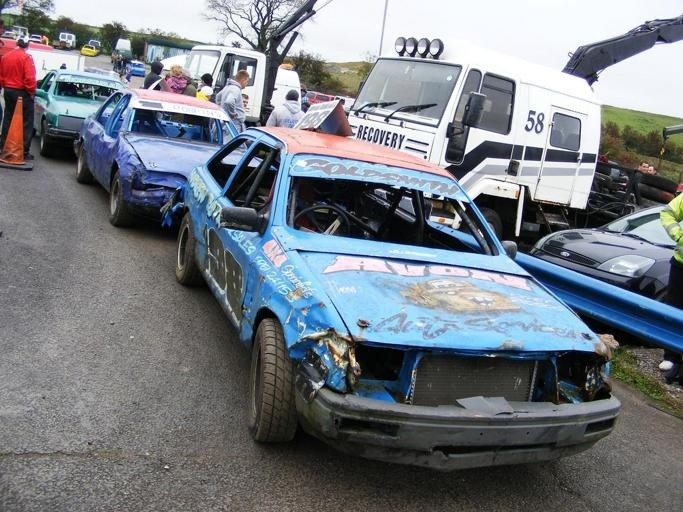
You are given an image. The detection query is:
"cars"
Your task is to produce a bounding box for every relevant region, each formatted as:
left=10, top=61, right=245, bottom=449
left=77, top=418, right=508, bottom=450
left=164, top=129, right=624, bottom=476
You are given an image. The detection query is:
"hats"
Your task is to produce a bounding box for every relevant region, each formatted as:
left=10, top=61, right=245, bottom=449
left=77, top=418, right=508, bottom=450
left=16, top=35, right=29, bottom=44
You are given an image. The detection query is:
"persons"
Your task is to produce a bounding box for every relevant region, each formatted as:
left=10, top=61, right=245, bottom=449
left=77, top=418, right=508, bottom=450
left=241, top=94, right=249, bottom=107
left=1, top=35, right=35, bottom=163
left=144, top=61, right=215, bottom=101
left=112, top=49, right=132, bottom=89
left=40, top=35, right=47, bottom=44
left=647, top=165, right=657, bottom=176
left=637, top=161, right=649, bottom=175
left=265, top=91, right=304, bottom=129
left=659, top=193, right=683, bottom=370
left=216, top=71, right=249, bottom=134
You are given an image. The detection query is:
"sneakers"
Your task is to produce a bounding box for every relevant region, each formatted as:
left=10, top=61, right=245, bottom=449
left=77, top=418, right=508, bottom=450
left=659, top=360, right=675, bottom=371
left=24, top=154, right=34, bottom=160
